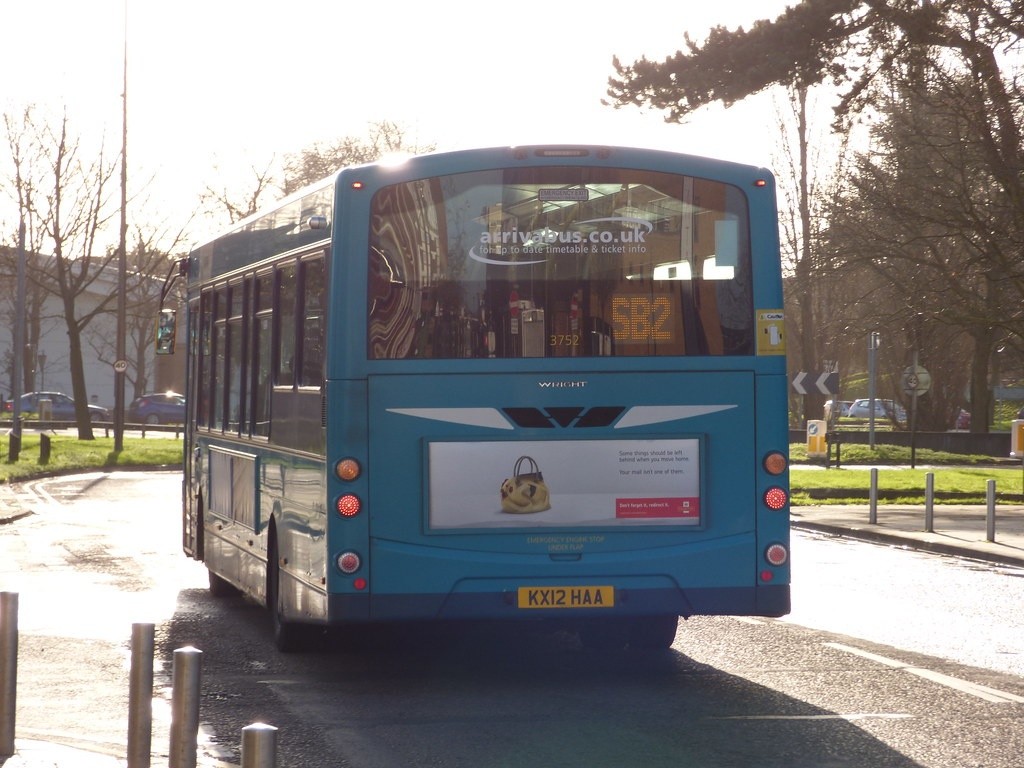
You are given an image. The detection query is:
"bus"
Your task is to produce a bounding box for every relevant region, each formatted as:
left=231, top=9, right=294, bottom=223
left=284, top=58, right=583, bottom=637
left=155, top=143, right=791, bottom=648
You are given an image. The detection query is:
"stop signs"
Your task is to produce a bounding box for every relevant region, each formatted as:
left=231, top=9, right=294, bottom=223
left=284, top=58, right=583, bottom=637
left=568, top=295, right=579, bottom=319
left=509, top=290, right=520, bottom=316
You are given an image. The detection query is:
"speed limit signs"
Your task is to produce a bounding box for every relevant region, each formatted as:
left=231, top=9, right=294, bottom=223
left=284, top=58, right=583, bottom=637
left=906, top=374, right=918, bottom=388
left=114, top=360, right=128, bottom=372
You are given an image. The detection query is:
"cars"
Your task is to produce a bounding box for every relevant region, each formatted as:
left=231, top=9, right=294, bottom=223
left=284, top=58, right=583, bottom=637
left=128, top=391, right=187, bottom=425
left=949, top=408, right=972, bottom=430
left=826, top=401, right=854, bottom=417
left=6, top=391, right=110, bottom=422
left=850, top=399, right=906, bottom=421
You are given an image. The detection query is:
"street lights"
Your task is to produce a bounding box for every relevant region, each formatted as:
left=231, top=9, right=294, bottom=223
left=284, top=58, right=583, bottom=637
left=38, top=351, right=47, bottom=390
